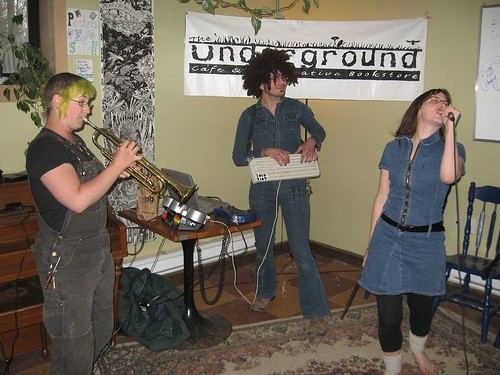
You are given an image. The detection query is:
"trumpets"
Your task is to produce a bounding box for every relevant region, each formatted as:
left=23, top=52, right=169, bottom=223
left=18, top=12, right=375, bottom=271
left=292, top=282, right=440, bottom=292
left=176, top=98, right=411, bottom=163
left=82, top=116, right=198, bottom=207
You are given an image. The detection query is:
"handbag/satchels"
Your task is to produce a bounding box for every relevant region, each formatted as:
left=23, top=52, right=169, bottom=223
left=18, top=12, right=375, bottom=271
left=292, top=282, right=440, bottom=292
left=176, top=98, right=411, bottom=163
left=117, top=267, right=189, bottom=352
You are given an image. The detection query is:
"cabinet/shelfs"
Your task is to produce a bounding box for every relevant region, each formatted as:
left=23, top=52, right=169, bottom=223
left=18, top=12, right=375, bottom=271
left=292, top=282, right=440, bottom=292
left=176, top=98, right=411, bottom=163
left=1, top=173, right=125, bottom=358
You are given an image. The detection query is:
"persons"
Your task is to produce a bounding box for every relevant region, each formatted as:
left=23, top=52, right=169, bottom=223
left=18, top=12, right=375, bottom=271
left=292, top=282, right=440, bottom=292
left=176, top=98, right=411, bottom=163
left=26, top=72, right=143, bottom=375
left=356, top=88, right=466, bottom=375
left=231, top=47, right=331, bottom=338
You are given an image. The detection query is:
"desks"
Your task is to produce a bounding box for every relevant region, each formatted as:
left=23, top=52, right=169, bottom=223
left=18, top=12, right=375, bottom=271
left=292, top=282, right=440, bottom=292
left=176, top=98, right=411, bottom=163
left=119, top=203, right=261, bottom=348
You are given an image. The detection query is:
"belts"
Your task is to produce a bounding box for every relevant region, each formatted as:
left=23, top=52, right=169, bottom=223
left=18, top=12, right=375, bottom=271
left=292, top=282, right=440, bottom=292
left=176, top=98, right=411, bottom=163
left=380, top=212, right=446, bottom=232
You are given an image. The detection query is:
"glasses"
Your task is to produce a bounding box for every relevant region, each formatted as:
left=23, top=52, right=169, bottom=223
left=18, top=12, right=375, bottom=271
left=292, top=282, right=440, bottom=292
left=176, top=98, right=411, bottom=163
left=270, top=73, right=288, bottom=81
left=49, top=94, right=93, bottom=108
left=428, top=96, right=449, bottom=107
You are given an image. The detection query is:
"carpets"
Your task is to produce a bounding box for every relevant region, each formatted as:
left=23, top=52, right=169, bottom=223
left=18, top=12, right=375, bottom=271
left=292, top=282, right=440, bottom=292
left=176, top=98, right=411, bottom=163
left=97, top=299, right=500, bottom=375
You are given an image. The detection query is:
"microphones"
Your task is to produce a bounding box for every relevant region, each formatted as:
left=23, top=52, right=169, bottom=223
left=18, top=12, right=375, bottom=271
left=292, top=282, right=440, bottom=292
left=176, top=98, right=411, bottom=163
left=447, top=112, right=455, bottom=122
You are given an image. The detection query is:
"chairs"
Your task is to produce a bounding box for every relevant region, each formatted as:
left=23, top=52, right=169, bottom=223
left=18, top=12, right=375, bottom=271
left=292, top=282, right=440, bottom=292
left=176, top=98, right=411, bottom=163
left=438, top=183, right=500, bottom=343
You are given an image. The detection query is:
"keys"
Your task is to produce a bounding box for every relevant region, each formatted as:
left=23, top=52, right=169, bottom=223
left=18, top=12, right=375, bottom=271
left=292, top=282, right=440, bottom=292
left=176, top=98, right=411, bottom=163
left=305, top=181, right=313, bottom=195
left=44, top=272, right=56, bottom=290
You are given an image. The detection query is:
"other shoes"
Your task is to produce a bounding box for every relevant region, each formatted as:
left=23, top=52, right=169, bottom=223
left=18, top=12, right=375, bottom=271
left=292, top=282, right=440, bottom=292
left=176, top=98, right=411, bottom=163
left=249, top=294, right=276, bottom=312
left=307, top=316, right=328, bottom=336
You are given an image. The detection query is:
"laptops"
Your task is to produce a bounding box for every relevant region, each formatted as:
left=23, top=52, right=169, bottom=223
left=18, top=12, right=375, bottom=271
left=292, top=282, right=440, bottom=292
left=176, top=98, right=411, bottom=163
left=161, top=168, right=228, bottom=215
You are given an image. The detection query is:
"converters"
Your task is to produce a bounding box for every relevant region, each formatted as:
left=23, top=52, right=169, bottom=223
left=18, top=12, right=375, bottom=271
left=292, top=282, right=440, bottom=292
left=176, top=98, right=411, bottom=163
left=6, top=202, right=23, bottom=210
